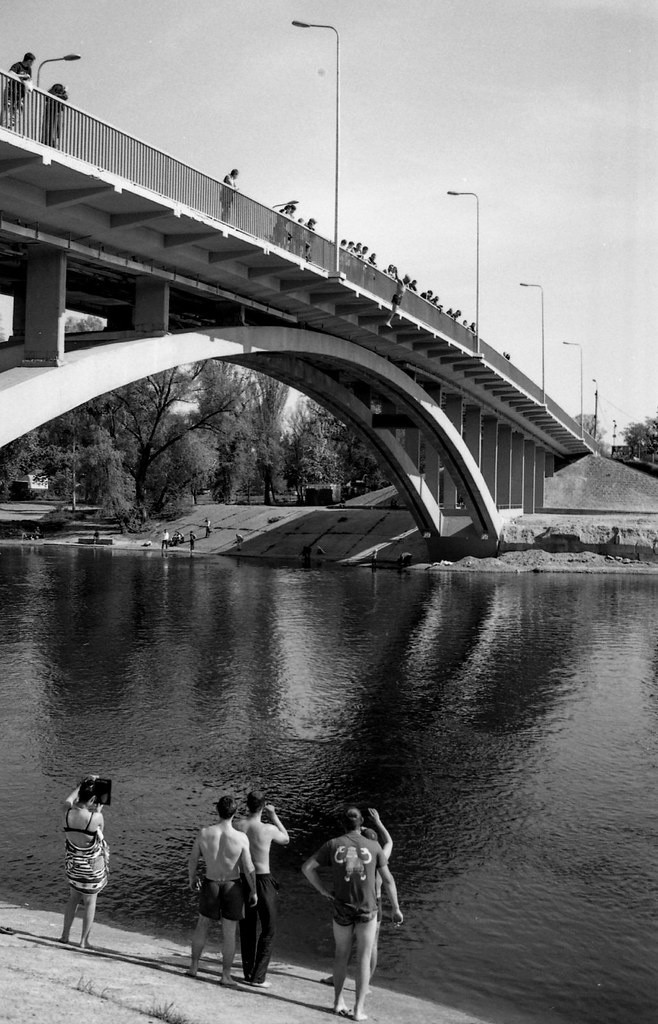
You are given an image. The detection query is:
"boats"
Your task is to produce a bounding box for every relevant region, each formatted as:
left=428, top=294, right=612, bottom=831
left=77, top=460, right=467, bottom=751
left=78, top=538, right=112, bottom=545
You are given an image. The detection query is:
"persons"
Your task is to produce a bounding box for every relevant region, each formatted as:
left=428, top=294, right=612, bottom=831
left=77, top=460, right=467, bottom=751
left=59, top=774, right=109, bottom=948
left=218, top=168, right=240, bottom=222
left=301, top=805, right=404, bottom=1022
left=236, top=534, right=244, bottom=551
left=41, top=83, right=69, bottom=148
left=189, top=790, right=291, bottom=988
left=274, top=204, right=317, bottom=263
left=399, top=552, right=413, bottom=567
left=340, top=238, right=476, bottom=334
left=160, top=517, right=213, bottom=550
left=302, top=544, right=326, bottom=560
left=93, top=529, right=100, bottom=545
left=22, top=526, right=41, bottom=541
left=371, top=548, right=378, bottom=575
left=0, top=52, right=36, bottom=129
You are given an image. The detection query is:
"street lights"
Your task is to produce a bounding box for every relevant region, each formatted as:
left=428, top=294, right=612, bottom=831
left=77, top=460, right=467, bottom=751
left=520, top=283, right=545, bottom=404
left=272, top=200, right=299, bottom=209
left=447, top=191, right=480, bottom=353
left=36, top=54, right=81, bottom=88
left=593, top=378, right=598, bottom=438
left=292, top=21, right=340, bottom=272
left=562, top=341, right=584, bottom=439
